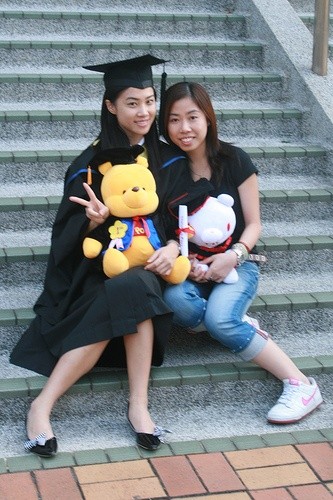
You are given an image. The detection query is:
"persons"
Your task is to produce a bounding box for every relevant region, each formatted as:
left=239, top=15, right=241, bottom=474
left=158, top=81, right=323, bottom=424
left=10, top=54, right=187, bottom=457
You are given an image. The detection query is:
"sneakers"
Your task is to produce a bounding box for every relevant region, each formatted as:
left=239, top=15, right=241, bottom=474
left=267, top=378, right=323, bottom=423
left=242, top=315, right=260, bottom=329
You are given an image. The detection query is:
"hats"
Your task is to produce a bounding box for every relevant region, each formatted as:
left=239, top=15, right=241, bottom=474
left=82, top=54, right=171, bottom=134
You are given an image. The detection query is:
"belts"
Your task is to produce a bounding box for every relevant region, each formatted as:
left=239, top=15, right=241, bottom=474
left=246, top=254, right=266, bottom=262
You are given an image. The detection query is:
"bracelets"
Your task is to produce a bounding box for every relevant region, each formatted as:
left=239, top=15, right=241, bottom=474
left=233, top=242, right=250, bottom=261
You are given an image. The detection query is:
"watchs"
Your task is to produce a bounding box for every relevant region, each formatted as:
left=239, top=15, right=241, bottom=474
left=232, top=248, right=244, bottom=266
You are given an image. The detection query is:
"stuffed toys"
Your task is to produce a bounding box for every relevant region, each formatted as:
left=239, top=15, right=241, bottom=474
left=83, top=156, right=191, bottom=283
left=172, top=178, right=238, bottom=283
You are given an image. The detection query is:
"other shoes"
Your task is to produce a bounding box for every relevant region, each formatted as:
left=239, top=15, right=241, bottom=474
left=24, top=414, right=57, bottom=458
left=126, top=405, right=160, bottom=450
